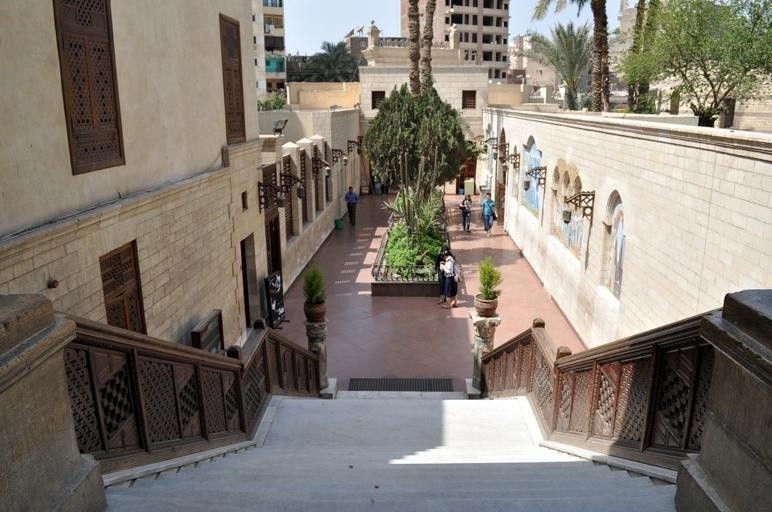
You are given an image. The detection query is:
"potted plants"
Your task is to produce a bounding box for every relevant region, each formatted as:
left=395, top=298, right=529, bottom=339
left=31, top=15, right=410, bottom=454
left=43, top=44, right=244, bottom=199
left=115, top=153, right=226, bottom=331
left=473, top=256, right=502, bottom=316
left=303, top=266, right=328, bottom=322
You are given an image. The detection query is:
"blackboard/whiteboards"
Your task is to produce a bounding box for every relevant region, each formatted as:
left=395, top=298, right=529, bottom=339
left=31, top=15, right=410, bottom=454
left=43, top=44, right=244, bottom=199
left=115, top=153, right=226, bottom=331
left=264, top=272, right=286, bottom=327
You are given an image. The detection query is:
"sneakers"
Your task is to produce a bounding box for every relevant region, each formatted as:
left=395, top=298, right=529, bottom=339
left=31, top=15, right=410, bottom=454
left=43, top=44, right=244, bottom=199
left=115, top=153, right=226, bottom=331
left=436, top=299, right=456, bottom=308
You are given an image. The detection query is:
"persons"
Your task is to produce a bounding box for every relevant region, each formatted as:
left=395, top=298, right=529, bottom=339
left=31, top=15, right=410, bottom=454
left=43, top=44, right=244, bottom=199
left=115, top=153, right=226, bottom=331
left=459, top=193, right=473, bottom=232
left=434, top=244, right=460, bottom=308
left=481, top=192, right=498, bottom=237
left=345, top=186, right=359, bottom=226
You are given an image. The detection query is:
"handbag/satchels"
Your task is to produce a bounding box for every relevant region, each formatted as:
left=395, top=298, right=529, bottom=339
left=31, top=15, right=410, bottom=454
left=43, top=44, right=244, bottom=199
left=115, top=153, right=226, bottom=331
left=492, top=212, right=496, bottom=220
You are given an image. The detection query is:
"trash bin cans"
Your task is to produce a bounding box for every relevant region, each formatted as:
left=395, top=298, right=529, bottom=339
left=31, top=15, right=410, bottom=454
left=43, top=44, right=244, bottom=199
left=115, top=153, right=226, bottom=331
left=459, top=189, right=464, bottom=195
left=335, top=219, right=344, bottom=229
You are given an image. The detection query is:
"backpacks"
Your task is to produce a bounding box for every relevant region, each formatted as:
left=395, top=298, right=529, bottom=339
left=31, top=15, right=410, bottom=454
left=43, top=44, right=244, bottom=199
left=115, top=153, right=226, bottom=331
left=446, top=259, right=462, bottom=283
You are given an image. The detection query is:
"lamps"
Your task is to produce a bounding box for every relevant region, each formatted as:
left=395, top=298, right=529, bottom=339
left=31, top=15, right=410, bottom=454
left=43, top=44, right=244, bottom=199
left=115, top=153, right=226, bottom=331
left=484, top=137, right=521, bottom=171
left=313, top=158, right=331, bottom=177
left=524, top=165, right=547, bottom=192
left=331, top=140, right=363, bottom=165
left=562, top=188, right=596, bottom=226
left=256, top=172, right=306, bottom=214
